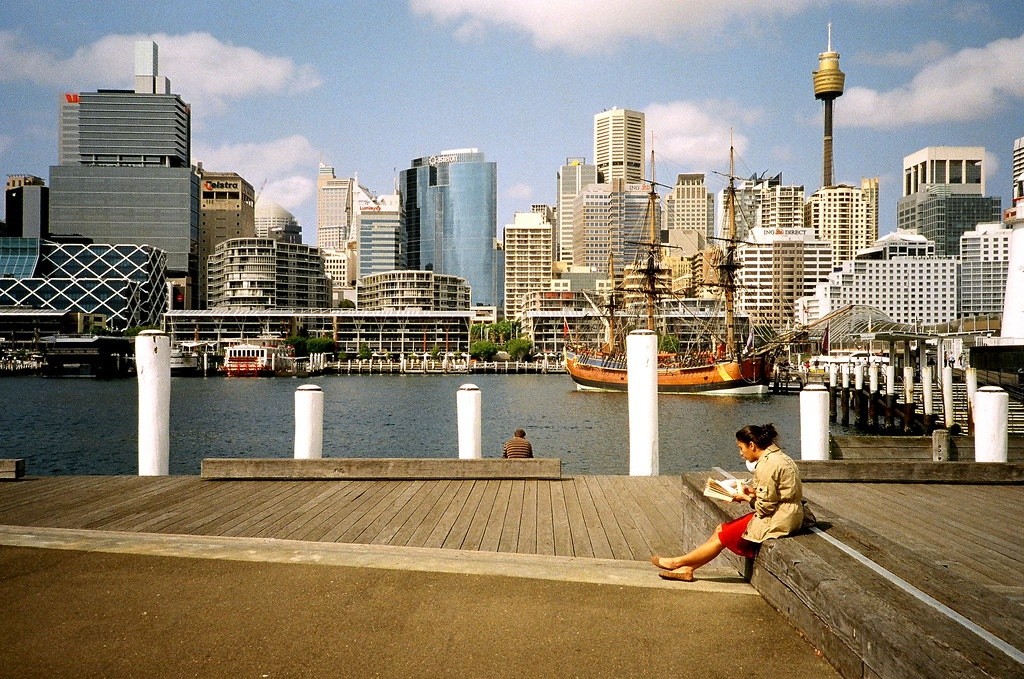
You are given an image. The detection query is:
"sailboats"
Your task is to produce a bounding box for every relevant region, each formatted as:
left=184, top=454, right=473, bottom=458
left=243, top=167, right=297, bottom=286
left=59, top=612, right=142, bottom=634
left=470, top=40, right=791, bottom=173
left=565, top=127, right=855, bottom=397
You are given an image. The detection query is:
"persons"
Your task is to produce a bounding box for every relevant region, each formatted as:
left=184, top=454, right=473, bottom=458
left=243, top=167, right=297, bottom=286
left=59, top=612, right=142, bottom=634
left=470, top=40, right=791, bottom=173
left=944, top=350, right=955, bottom=369
left=958, top=353, right=966, bottom=367
left=804, top=359, right=819, bottom=373
left=502, top=428, right=534, bottom=458
left=651, top=423, right=804, bottom=581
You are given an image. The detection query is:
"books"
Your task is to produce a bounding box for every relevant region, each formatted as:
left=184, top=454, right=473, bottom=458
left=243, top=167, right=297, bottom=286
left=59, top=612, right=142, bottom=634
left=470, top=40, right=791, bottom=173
left=704, top=477, right=740, bottom=502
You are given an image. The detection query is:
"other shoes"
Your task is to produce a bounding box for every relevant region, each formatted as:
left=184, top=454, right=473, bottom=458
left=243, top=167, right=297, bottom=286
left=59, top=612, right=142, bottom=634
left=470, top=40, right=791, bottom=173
left=651, top=556, right=694, bottom=582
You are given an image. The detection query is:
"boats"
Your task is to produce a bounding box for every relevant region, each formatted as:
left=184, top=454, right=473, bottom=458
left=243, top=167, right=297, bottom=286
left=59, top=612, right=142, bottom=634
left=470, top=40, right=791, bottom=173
left=170, top=342, right=198, bottom=374
left=811, top=350, right=890, bottom=370
left=222, top=341, right=294, bottom=379
